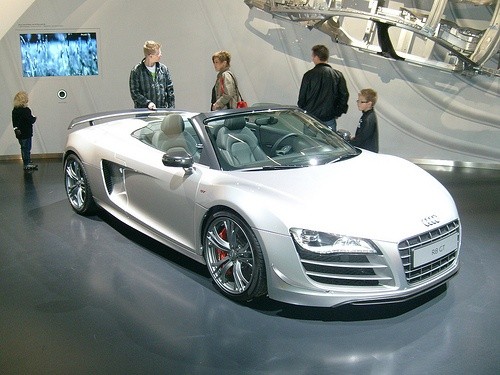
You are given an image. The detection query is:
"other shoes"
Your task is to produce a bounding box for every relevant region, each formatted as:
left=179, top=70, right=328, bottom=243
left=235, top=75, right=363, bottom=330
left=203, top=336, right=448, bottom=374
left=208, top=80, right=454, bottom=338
left=24, top=164, right=38, bottom=169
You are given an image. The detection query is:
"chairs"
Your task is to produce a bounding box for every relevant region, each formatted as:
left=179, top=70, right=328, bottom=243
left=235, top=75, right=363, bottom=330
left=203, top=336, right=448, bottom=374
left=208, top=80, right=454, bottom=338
left=214, top=117, right=268, bottom=166
left=152, top=114, right=200, bottom=163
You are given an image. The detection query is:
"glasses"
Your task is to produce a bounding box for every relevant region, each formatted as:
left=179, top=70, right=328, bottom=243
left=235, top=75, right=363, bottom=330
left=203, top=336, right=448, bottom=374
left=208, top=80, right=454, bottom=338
left=357, top=100, right=368, bottom=104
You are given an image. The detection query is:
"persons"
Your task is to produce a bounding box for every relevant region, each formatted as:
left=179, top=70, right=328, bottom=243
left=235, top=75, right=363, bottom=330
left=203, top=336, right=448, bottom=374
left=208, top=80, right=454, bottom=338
left=11, top=91, right=39, bottom=170
left=296, top=45, right=349, bottom=139
left=211, top=50, right=240, bottom=111
left=129, top=41, right=175, bottom=117
left=350, top=88, right=379, bottom=153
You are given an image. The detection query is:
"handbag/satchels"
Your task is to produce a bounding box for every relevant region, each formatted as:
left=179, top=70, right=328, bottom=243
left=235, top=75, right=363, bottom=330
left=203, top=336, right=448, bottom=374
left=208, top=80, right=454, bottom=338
left=237, top=101, right=247, bottom=109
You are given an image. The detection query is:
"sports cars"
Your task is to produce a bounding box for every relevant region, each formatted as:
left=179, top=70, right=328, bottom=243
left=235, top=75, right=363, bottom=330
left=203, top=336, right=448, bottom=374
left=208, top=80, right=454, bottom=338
left=62, top=100, right=464, bottom=312
